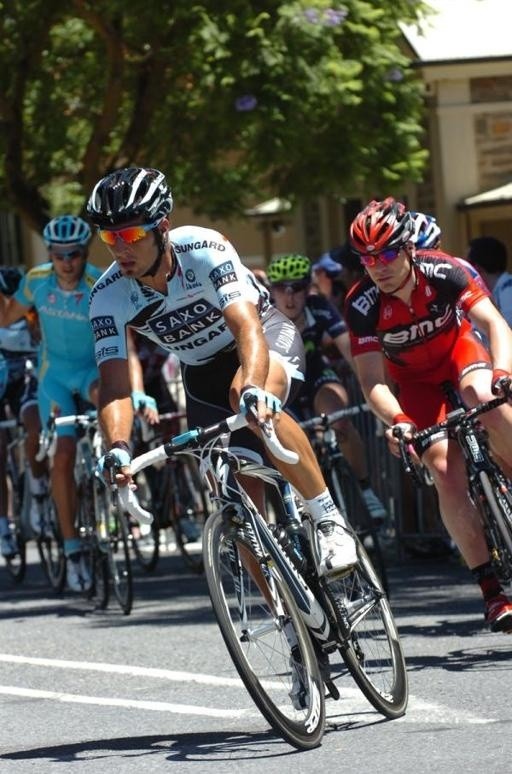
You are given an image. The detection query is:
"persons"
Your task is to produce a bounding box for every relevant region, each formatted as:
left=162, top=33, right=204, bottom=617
left=248, top=243, right=390, bottom=521
left=77, top=164, right=361, bottom=709
left=0, top=215, right=201, bottom=590
left=344, top=197, right=512, bottom=623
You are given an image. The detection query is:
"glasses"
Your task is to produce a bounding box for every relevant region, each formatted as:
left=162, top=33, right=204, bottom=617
left=97, top=215, right=167, bottom=245
left=53, top=250, right=80, bottom=261
left=360, top=244, right=403, bottom=266
left=272, top=282, right=308, bottom=293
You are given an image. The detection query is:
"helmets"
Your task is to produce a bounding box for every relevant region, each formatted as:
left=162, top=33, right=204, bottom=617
left=43, top=216, right=91, bottom=251
left=87, top=168, right=173, bottom=222
left=0, top=265, right=29, bottom=294
left=267, top=256, right=312, bottom=283
left=349, top=197, right=441, bottom=256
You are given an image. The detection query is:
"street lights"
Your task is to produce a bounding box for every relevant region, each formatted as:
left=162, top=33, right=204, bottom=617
left=245, top=195, right=295, bottom=269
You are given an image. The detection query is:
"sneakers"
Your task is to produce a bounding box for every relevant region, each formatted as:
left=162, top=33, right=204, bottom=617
left=2, top=529, right=19, bottom=557
left=362, top=490, right=388, bottom=523
left=485, top=595, right=511, bottom=633
left=318, top=521, right=358, bottom=577
left=290, top=648, right=311, bottom=709
left=66, top=550, right=93, bottom=593
left=177, top=515, right=201, bottom=540
left=29, top=493, right=56, bottom=538
